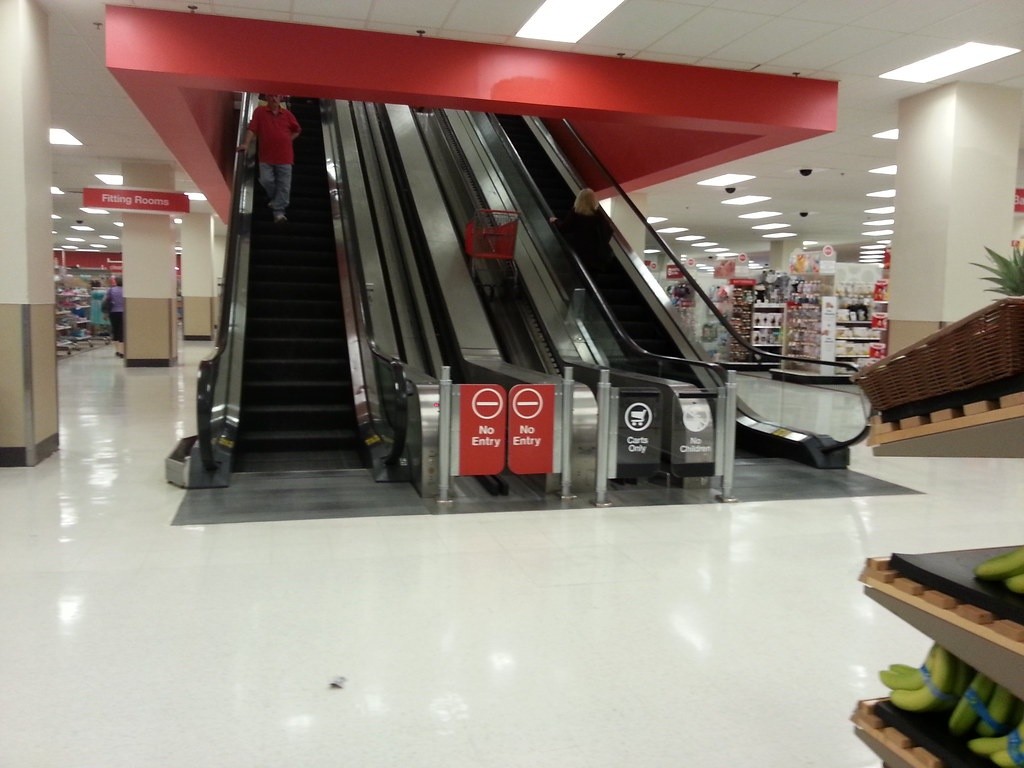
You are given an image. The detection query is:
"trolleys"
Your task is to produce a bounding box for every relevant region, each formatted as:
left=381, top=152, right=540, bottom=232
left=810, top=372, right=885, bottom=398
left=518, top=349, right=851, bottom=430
left=463, top=208, right=521, bottom=298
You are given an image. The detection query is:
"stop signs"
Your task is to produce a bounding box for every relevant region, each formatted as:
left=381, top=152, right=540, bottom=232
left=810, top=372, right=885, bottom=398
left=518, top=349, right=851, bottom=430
left=738, top=252, right=747, bottom=264
left=822, top=245, right=832, bottom=258
left=470, top=388, right=504, bottom=420
left=688, top=258, right=696, bottom=267
left=512, top=387, right=543, bottom=420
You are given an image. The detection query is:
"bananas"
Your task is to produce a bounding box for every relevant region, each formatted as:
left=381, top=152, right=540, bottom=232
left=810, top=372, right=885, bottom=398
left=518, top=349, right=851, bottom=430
left=879, top=646, right=1024, bottom=768
left=974, top=546, right=1024, bottom=593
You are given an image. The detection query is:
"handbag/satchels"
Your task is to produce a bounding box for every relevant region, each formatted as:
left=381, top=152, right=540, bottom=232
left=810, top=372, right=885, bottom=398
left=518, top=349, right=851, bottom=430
left=101, top=288, right=114, bottom=313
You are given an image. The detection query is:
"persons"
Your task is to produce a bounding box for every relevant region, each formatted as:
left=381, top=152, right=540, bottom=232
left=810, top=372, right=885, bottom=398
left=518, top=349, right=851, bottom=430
left=88, top=279, right=111, bottom=346
left=70, top=297, right=87, bottom=346
left=235, top=92, right=303, bottom=226
left=104, top=275, right=125, bottom=358
left=549, top=188, right=614, bottom=320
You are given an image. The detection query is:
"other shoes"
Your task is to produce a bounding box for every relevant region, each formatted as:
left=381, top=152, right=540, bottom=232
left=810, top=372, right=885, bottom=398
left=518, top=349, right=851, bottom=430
left=116, top=352, right=120, bottom=356
left=275, top=215, right=287, bottom=223
left=120, top=354, right=124, bottom=358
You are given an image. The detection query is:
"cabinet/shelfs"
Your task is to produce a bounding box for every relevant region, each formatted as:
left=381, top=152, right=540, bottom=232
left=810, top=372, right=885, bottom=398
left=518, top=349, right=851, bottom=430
left=850, top=543, right=1024, bottom=768
left=665, top=270, right=890, bottom=385
left=56, top=286, right=112, bottom=356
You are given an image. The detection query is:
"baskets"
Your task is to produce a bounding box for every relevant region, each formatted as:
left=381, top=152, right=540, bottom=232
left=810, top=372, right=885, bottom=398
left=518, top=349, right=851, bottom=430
left=849, top=297, right=1024, bottom=412
